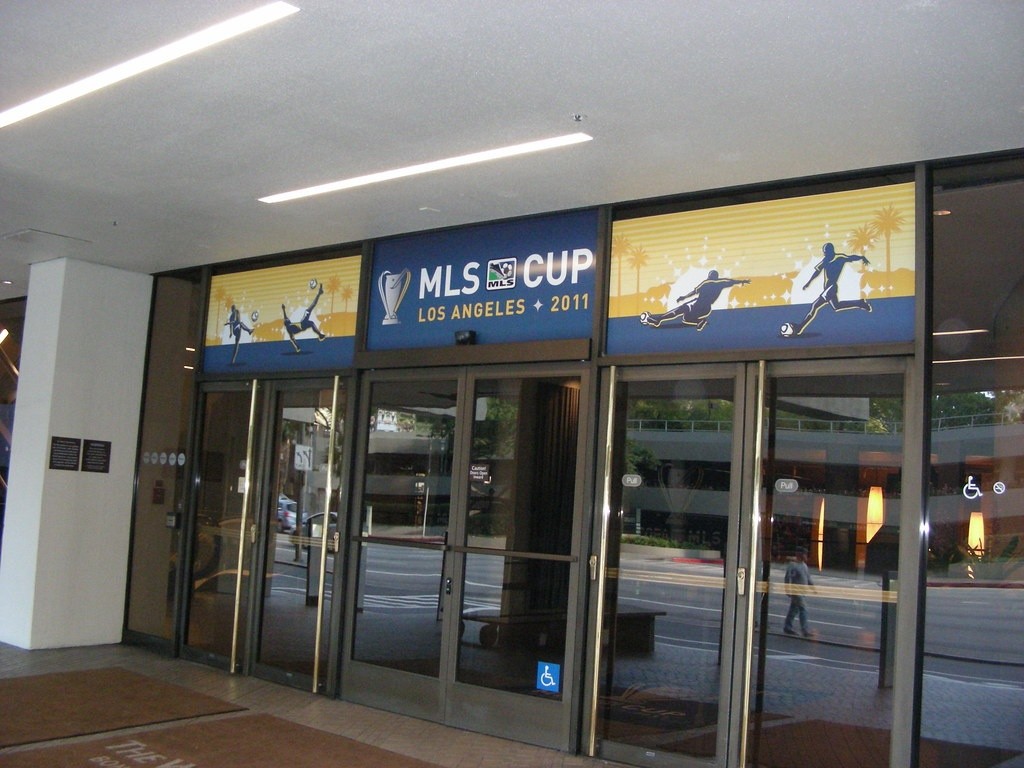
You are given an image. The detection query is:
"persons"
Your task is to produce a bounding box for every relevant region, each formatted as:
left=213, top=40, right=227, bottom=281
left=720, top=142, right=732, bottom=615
left=782, top=549, right=818, bottom=636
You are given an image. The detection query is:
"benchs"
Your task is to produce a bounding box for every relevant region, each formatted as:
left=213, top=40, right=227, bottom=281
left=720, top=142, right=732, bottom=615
left=460, top=600, right=666, bottom=655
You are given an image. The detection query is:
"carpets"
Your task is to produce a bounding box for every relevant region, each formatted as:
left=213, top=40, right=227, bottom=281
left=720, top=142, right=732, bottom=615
left=658, top=719, right=1023, bottom=768
left=0, top=667, right=250, bottom=748
left=490, top=683, right=796, bottom=739
left=0, top=713, right=449, bottom=768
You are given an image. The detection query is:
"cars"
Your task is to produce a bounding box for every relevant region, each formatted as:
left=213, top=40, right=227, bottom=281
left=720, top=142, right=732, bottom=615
left=166, top=495, right=338, bottom=662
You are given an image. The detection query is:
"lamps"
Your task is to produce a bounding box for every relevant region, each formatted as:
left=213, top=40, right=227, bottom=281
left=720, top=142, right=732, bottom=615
left=455, top=330, right=477, bottom=346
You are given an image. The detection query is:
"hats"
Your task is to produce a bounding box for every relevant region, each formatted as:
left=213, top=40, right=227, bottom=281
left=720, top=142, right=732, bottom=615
left=795, top=546, right=808, bottom=553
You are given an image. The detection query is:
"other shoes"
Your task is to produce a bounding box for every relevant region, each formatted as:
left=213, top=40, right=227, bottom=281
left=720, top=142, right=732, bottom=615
left=803, top=632, right=820, bottom=640
left=784, top=630, right=801, bottom=636
